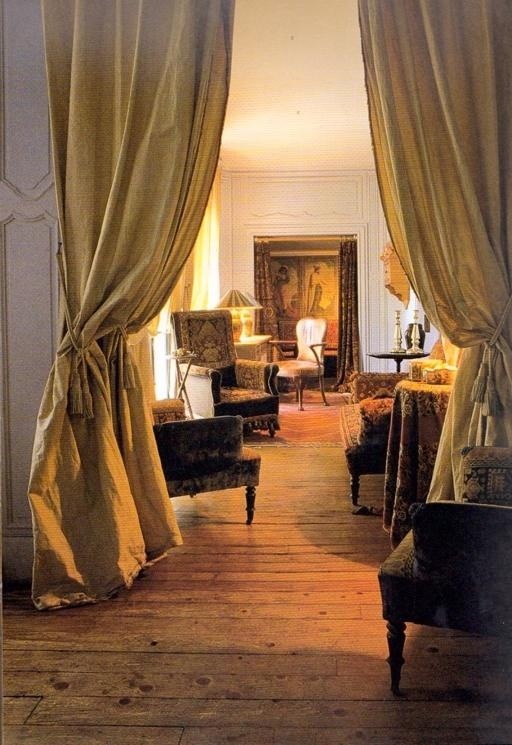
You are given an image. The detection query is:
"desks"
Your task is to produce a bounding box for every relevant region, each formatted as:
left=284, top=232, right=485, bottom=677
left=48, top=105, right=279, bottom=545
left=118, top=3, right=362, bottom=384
left=368, top=349, right=431, bottom=375
left=233, top=334, right=272, bottom=361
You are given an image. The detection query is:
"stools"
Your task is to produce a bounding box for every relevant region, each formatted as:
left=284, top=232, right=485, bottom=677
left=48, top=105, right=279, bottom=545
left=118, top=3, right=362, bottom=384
left=154, top=399, right=184, bottom=426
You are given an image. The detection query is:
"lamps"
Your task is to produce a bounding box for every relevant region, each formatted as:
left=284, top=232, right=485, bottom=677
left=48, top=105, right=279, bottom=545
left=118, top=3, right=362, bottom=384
left=213, top=287, right=262, bottom=343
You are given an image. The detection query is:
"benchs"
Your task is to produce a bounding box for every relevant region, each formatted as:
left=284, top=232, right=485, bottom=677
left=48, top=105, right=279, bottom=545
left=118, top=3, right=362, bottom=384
left=338, top=367, right=459, bottom=506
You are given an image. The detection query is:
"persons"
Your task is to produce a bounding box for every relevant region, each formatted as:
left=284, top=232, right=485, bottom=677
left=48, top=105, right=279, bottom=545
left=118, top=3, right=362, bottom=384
left=307, top=266, right=325, bottom=313
left=273, top=265, right=295, bottom=316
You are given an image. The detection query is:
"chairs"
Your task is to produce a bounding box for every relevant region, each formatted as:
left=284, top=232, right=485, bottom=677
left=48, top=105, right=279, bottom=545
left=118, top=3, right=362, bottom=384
left=151, top=415, right=261, bottom=525
left=265, top=317, right=329, bottom=411
left=169, top=308, right=280, bottom=442
left=361, top=447, right=511, bottom=696
